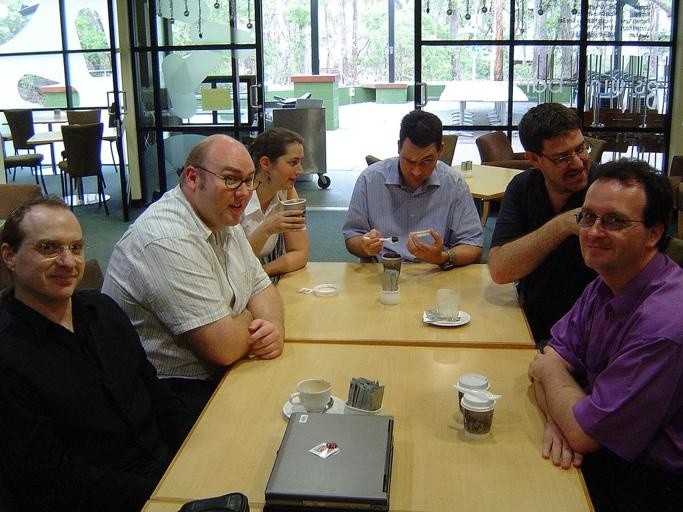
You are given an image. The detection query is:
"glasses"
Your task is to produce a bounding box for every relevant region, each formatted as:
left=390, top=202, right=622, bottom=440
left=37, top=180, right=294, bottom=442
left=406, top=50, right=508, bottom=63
left=194, top=165, right=261, bottom=192
left=574, top=210, right=643, bottom=231
left=541, top=138, right=592, bottom=165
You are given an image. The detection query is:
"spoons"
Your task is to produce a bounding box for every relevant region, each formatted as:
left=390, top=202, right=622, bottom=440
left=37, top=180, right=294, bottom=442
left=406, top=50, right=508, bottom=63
left=451, top=383, right=502, bottom=401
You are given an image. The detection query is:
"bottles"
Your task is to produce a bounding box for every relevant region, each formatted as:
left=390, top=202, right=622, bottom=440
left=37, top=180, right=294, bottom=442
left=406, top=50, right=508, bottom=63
left=459, top=161, right=472, bottom=178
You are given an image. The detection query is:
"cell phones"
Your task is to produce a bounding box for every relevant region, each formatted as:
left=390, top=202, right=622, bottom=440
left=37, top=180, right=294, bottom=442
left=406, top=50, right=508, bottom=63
left=177, top=492, right=248, bottom=512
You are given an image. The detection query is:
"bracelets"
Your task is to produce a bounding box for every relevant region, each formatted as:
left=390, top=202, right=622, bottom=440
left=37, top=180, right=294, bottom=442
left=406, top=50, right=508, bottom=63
left=439, top=250, right=455, bottom=271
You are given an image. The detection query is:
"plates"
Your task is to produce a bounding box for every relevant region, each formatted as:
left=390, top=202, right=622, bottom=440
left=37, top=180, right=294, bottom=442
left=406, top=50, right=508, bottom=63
left=422, top=308, right=470, bottom=327
left=282, top=395, right=345, bottom=420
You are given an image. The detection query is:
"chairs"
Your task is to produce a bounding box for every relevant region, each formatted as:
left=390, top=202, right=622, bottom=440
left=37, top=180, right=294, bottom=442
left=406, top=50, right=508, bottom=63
left=534, top=55, right=669, bottom=169
left=0, top=108, right=109, bottom=217
left=364, top=130, right=607, bottom=214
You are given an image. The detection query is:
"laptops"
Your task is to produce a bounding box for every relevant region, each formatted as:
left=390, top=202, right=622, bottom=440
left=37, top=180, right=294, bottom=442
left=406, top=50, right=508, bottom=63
left=263, top=412, right=394, bottom=511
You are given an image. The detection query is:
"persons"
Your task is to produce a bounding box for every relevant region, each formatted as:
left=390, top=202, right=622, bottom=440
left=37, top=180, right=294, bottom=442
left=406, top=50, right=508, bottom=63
left=344, top=109, right=487, bottom=270
left=237, top=125, right=313, bottom=290
left=488, top=100, right=607, bottom=352
left=102, top=132, right=287, bottom=421
left=528, top=155, right=683, bottom=512
left=2, top=193, right=200, bottom=510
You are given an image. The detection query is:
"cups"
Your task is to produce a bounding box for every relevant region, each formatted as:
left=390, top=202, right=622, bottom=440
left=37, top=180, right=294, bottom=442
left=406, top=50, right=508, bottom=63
left=381, top=253, right=401, bottom=280
left=288, top=379, right=332, bottom=414
left=282, top=199, right=306, bottom=232
left=455, top=374, right=491, bottom=424
left=343, top=402, right=383, bottom=415
left=435, top=289, right=461, bottom=320
left=459, top=390, right=496, bottom=439
left=380, top=287, right=400, bottom=305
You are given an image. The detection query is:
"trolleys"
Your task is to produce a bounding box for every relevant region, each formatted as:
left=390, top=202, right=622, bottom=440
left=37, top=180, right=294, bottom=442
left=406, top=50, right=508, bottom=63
left=261, top=103, right=331, bottom=189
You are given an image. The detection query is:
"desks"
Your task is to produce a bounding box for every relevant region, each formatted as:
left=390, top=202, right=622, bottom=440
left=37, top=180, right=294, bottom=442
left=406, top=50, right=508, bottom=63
left=25, top=127, right=118, bottom=174
left=447, top=164, right=527, bottom=226
left=438, top=79, right=529, bottom=140
